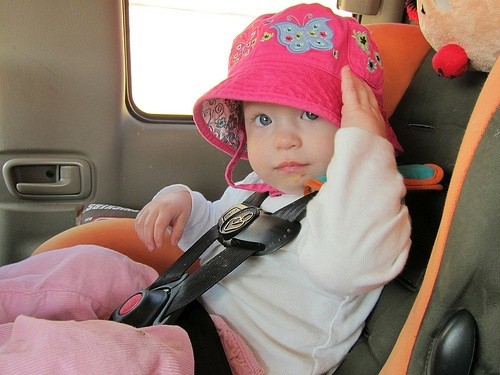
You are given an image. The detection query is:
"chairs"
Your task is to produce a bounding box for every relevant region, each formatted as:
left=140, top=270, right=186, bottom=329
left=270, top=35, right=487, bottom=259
left=29, top=22, right=500, bottom=375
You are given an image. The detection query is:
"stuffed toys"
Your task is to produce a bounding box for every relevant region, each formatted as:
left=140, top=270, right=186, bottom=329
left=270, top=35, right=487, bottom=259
left=406, top=0, right=500, bottom=78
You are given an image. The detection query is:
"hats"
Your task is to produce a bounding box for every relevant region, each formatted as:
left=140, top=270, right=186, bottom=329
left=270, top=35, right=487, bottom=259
left=193, top=3, right=405, bottom=161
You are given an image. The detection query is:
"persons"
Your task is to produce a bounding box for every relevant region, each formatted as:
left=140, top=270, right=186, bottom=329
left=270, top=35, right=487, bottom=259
left=0, top=3, right=411, bottom=374
